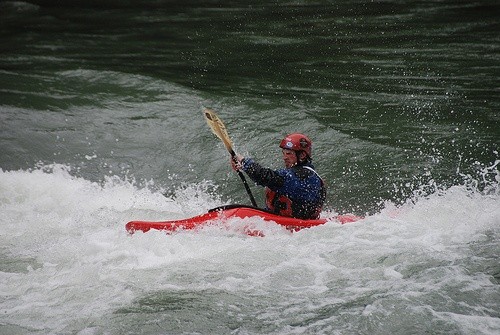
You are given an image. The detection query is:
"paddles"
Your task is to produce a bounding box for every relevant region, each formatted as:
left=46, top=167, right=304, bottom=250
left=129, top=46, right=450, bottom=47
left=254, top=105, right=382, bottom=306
left=200, top=105, right=258, bottom=209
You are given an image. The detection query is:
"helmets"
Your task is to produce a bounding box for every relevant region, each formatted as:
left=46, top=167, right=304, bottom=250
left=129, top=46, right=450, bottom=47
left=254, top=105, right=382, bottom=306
left=279, top=134, right=311, bottom=157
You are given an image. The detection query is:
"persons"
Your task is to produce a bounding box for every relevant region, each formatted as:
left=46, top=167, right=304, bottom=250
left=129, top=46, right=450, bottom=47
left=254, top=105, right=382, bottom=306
left=230, top=133, right=327, bottom=223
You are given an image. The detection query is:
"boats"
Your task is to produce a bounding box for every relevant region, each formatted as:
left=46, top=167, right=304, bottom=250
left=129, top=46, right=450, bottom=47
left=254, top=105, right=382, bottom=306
left=126, top=203, right=360, bottom=238
